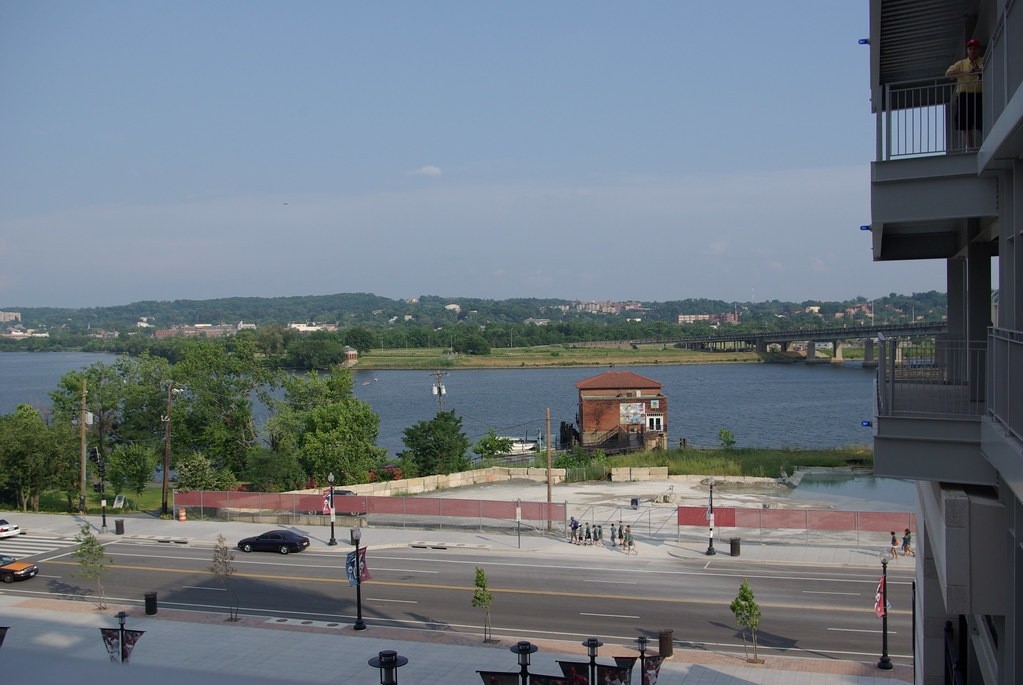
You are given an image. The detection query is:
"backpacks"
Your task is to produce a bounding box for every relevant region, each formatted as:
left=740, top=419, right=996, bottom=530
left=572, top=520, right=578, bottom=529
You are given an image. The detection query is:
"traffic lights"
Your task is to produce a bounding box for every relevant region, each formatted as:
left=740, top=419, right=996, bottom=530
left=89, top=448, right=99, bottom=464
left=98, top=463, right=106, bottom=477
left=95, top=483, right=101, bottom=493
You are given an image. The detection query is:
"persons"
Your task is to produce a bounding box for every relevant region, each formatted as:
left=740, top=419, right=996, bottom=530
left=901, top=528, right=915, bottom=557
left=946, top=39, right=983, bottom=151
left=891, top=531, right=898, bottom=559
left=569, top=516, right=638, bottom=555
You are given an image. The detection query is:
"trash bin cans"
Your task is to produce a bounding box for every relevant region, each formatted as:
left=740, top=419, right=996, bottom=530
left=144, top=591, right=158, bottom=615
left=115, top=519, right=124, bottom=535
left=658, top=629, right=674, bottom=657
left=350, top=529, right=360, bottom=545
left=730, top=537, right=741, bottom=557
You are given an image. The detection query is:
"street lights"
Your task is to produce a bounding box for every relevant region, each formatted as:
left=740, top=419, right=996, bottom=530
left=706, top=475, right=715, bottom=554
left=327, top=473, right=338, bottom=546
left=878, top=546, right=894, bottom=669
left=162, top=388, right=185, bottom=513
left=352, top=525, right=366, bottom=631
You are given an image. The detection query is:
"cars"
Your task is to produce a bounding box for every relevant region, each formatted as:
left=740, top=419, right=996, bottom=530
left=0, top=553, right=40, bottom=584
left=238, top=529, right=311, bottom=554
left=302, top=490, right=376, bottom=516
left=0, top=518, right=20, bottom=539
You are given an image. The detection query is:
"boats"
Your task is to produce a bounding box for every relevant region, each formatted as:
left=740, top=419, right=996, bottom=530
left=496, top=435, right=556, bottom=455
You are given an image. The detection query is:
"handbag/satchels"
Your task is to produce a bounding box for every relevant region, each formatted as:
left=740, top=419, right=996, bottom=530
left=895, top=538, right=900, bottom=545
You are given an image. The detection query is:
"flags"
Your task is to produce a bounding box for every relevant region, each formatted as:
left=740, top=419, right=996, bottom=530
left=323, top=489, right=334, bottom=514
left=346, top=550, right=358, bottom=587
left=359, top=547, right=372, bottom=583
left=875, top=575, right=892, bottom=618
